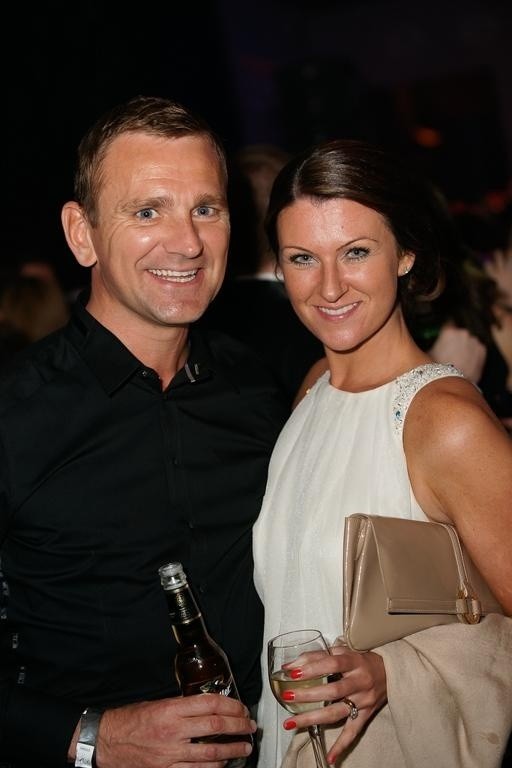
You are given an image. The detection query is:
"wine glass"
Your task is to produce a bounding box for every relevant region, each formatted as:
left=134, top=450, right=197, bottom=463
left=268, top=631, right=336, bottom=768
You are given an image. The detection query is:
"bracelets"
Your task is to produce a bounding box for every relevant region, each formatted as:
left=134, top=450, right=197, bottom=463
left=74, top=706, right=104, bottom=768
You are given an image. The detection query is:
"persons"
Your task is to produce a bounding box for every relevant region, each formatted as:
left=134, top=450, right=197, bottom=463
left=0, top=98, right=304, bottom=768
left=426, top=213, right=512, bottom=434
left=207, top=139, right=324, bottom=357
left=251, top=139, right=512, bottom=768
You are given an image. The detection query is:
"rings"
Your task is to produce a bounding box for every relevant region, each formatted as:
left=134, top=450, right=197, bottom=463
left=340, top=698, right=358, bottom=719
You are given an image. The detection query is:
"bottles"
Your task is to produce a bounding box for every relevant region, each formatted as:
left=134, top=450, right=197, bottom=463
left=159, top=563, right=254, bottom=759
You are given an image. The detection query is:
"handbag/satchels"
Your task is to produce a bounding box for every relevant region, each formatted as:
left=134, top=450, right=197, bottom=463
left=341, top=512, right=503, bottom=654
